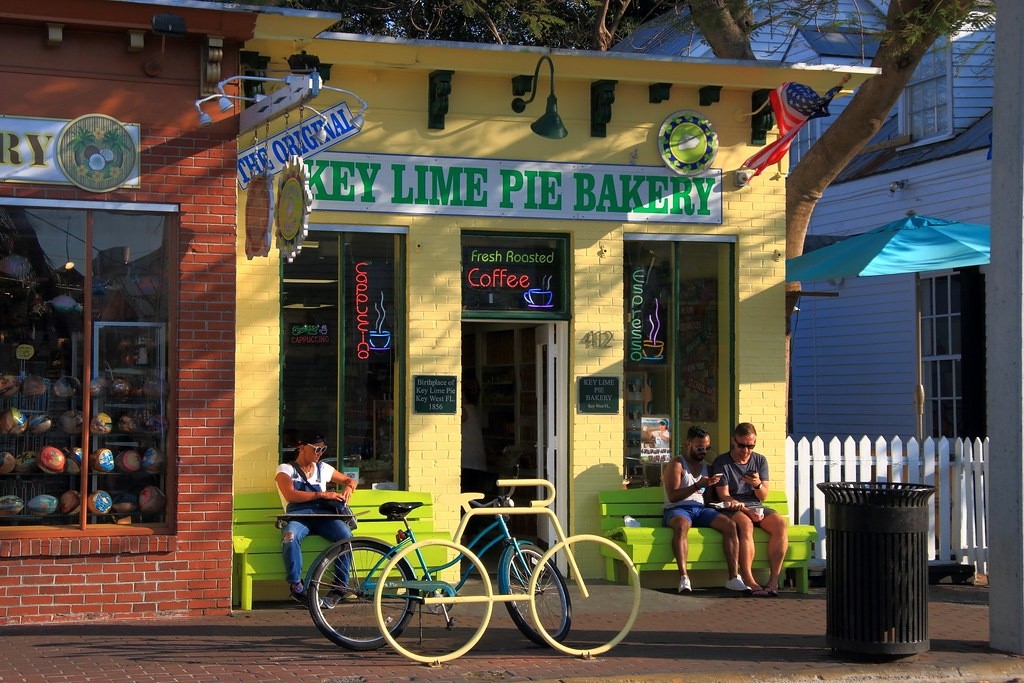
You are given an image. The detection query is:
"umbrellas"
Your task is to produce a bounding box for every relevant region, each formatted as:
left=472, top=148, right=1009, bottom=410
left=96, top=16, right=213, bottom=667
left=786, top=209, right=990, bottom=440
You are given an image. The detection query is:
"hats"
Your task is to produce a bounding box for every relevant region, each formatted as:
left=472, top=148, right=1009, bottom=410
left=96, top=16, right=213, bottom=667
left=295, top=430, right=326, bottom=444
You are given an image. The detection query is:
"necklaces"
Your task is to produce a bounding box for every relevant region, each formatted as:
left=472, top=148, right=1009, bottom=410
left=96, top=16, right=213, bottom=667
left=299, top=465, right=312, bottom=475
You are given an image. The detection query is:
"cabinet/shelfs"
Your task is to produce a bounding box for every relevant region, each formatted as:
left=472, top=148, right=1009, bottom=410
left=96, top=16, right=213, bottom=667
left=0, top=321, right=166, bottom=517
left=466, top=328, right=538, bottom=544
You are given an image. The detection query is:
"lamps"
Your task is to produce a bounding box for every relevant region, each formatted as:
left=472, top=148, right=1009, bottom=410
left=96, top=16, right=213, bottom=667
left=195, top=93, right=264, bottom=127
left=512, top=55, right=568, bottom=140
left=888, top=180, right=905, bottom=196
left=322, top=86, right=367, bottom=131
left=151, top=10, right=188, bottom=38
left=302, top=105, right=327, bottom=144
left=217, top=74, right=286, bottom=112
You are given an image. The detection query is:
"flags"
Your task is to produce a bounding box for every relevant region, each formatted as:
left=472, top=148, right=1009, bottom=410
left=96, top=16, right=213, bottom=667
left=744, top=81, right=844, bottom=175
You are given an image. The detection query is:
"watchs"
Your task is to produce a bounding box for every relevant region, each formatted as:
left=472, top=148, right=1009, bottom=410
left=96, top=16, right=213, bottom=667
left=753, top=482, right=762, bottom=489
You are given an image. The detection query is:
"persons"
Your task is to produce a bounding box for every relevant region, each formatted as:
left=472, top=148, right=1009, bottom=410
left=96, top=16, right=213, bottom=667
left=276, top=432, right=358, bottom=608
left=462, top=368, right=487, bottom=490
left=647, top=420, right=669, bottom=448
left=662, top=426, right=752, bottom=594
left=712, top=422, right=788, bottom=597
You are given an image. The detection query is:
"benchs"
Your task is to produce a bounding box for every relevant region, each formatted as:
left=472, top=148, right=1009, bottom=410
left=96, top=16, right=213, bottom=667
left=231, top=489, right=450, bottom=612
left=596, top=486, right=820, bottom=595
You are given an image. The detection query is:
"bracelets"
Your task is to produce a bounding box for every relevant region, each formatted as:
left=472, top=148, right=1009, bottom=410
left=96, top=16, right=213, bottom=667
left=348, top=485, right=355, bottom=492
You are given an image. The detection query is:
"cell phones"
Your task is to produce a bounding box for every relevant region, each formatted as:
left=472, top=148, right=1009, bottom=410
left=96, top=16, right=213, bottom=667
left=741, top=470, right=758, bottom=481
left=713, top=474, right=723, bottom=478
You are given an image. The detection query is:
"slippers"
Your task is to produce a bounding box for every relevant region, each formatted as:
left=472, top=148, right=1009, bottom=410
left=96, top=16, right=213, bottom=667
left=765, top=585, right=777, bottom=596
left=751, top=589, right=766, bottom=597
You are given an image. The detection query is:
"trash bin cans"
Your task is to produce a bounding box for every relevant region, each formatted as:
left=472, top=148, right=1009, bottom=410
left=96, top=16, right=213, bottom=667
left=816, top=482, right=937, bottom=663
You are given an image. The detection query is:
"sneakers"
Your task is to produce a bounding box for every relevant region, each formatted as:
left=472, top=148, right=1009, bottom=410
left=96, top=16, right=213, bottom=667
left=725, top=574, right=752, bottom=592
left=289, top=579, right=323, bottom=609
left=323, top=587, right=342, bottom=608
left=678, top=575, right=692, bottom=593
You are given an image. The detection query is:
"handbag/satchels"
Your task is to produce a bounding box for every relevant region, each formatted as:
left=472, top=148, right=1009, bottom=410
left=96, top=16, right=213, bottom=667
left=316, top=497, right=357, bottom=530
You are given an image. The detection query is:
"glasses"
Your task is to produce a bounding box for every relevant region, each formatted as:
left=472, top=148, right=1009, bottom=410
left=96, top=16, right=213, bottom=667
left=734, top=437, right=756, bottom=449
left=307, top=443, right=327, bottom=454
left=692, top=443, right=712, bottom=452
